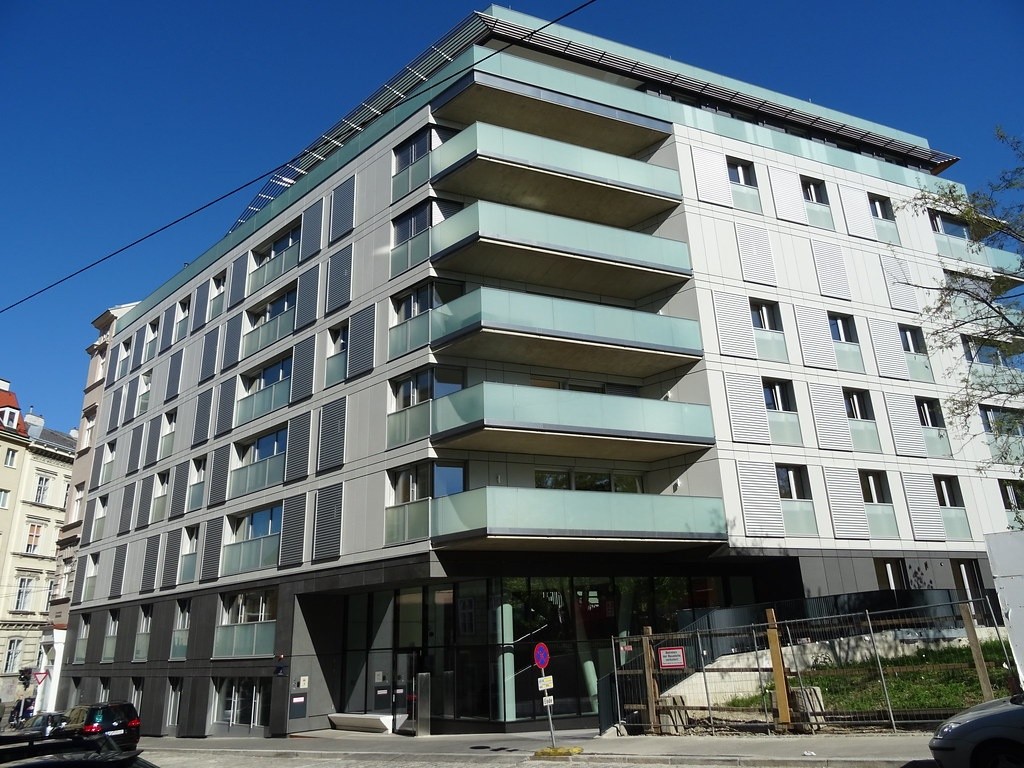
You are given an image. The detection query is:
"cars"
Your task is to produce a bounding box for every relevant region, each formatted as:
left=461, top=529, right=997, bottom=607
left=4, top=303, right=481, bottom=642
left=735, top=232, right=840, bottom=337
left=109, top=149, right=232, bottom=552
left=928, top=692, right=1024, bottom=768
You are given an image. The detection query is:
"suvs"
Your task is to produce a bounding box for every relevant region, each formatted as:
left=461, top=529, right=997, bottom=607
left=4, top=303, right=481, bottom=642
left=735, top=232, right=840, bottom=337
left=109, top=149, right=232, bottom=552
left=15, top=712, right=62, bottom=737
left=8, top=698, right=36, bottom=727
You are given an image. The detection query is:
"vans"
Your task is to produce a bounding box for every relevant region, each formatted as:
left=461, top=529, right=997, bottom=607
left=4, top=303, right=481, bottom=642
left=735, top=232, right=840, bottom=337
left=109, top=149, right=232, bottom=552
left=49, top=700, right=140, bottom=751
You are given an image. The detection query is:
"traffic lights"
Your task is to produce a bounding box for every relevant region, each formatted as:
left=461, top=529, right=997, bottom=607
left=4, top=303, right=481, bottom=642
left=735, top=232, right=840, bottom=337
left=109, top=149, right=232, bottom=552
left=24, top=668, right=33, bottom=687
left=19, top=670, right=26, bottom=682
